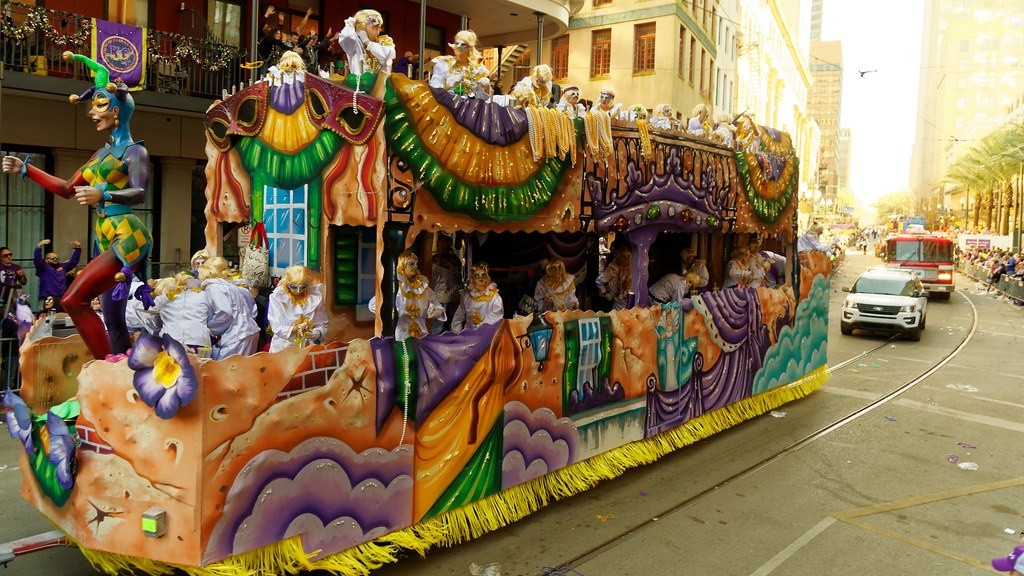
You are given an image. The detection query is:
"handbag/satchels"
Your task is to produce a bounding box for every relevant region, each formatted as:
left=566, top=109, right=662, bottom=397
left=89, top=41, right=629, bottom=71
left=242, top=221, right=270, bottom=287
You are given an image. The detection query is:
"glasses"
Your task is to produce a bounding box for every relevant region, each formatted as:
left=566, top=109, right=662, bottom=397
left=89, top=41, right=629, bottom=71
left=45, top=299, right=54, bottom=303
left=2, top=253, right=13, bottom=258
left=46, top=256, right=60, bottom=260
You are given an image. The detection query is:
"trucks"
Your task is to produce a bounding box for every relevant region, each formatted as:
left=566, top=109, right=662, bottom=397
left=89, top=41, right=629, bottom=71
left=883, top=235, right=955, bottom=301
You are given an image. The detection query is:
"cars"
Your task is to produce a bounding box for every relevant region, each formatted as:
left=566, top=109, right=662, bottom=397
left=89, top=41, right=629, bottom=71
left=840, top=268, right=928, bottom=341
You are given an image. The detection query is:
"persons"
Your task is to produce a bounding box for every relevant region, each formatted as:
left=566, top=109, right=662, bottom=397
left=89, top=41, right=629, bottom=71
left=0, top=239, right=102, bottom=318
left=367, top=251, right=447, bottom=340
left=797, top=222, right=836, bottom=252
left=2, top=52, right=154, bottom=359
left=629, top=100, right=647, bottom=121
left=451, top=264, right=504, bottom=333
left=857, top=230, right=1024, bottom=294
left=688, top=104, right=761, bottom=152
left=595, top=248, right=632, bottom=311
left=648, top=273, right=700, bottom=305
left=136, top=249, right=261, bottom=360
left=267, top=265, right=328, bottom=352
left=514, top=65, right=552, bottom=107
left=590, top=88, right=620, bottom=119
left=557, top=86, right=586, bottom=119
left=723, top=239, right=786, bottom=288
left=681, top=249, right=709, bottom=296
left=338, top=9, right=396, bottom=75
left=258, top=6, right=340, bottom=81
left=429, top=31, right=492, bottom=102
left=650, top=104, right=672, bottom=129
left=534, top=260, right=579, bottom=313
